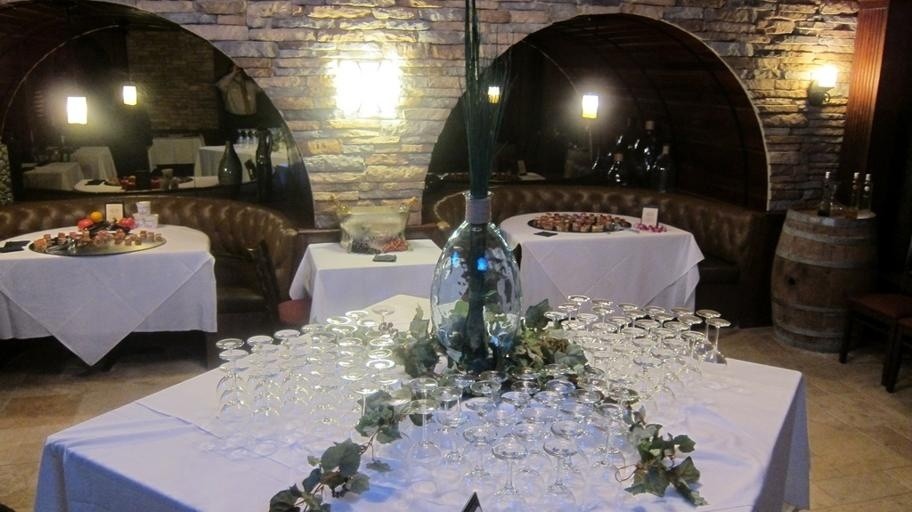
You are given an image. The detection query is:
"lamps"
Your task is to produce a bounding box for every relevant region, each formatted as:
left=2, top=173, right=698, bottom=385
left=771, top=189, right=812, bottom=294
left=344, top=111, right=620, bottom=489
left=806, top=70, right=834, bottom=106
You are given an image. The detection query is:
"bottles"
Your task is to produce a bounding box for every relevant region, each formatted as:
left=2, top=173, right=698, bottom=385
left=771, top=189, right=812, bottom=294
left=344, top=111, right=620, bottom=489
left=608, top=154, right=627, bottom=187
left=861, top=174, right=873, bottom=214
left=654, top=144, right=676, bottom=195
left=850, top=172, right=860, bottom=208
left=820, top=171, right=830, bottom=207
left=634, top=120, right=658, bottom=169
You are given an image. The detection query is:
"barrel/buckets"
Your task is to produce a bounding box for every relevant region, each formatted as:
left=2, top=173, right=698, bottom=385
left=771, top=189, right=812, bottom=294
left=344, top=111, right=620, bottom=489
left=770, top=207, right=877, bottom=354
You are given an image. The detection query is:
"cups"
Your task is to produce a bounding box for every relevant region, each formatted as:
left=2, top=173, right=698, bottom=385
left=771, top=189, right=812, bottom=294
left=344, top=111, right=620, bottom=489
left=159, top=178, right=169, bottom=189
left=143, top=213, right=159, bottom=229
left=162, top=168, right=173, bottom=177
left=170, top=177, right=180, bottom=189
left=136, top=201, right=151, bottom=216
left=132, top=213, right=144, bottom=228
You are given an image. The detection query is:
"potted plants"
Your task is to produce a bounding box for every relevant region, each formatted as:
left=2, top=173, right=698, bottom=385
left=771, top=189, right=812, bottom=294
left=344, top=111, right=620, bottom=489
left=435, top=0, right=519, bottom=374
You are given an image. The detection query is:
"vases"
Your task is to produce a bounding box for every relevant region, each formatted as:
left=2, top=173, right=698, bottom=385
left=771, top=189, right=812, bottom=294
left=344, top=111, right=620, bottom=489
left=218, top=141, right=243, bottom=184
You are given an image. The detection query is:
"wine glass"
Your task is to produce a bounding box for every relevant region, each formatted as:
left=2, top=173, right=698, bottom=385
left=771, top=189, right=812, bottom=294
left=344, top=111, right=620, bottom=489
left=251, top=129, right=258, bottom=147
left=237, top=129, right=245, bottom=148
left=244, top=129, right=251, bottom=148
left=217, top=303, right=399, bottom=456
left=543, top=292, right=732, bottom=438
left=407, top=367, right=627, bottom=511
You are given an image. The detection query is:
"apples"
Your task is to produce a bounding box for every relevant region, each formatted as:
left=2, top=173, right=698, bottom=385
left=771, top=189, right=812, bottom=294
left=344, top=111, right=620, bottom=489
left=78, top=219, right=93, bottom=231
left=119, top=217, right=136, bottom=230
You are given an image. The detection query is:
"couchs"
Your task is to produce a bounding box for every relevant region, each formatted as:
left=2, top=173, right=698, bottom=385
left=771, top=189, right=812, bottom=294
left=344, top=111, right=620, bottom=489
left=423, top=182, right=778, bottom=325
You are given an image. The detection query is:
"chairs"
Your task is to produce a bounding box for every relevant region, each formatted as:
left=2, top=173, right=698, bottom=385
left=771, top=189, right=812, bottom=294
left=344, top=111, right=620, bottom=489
left=244, top=239, right=309, bottom=332
left=888, top=318, right=912, bottom=392
left=696, top=207, right=787, bottom=329
left=842, top=238, right=912, bottom=385
left=0, top=195, right=314, bottom=309
